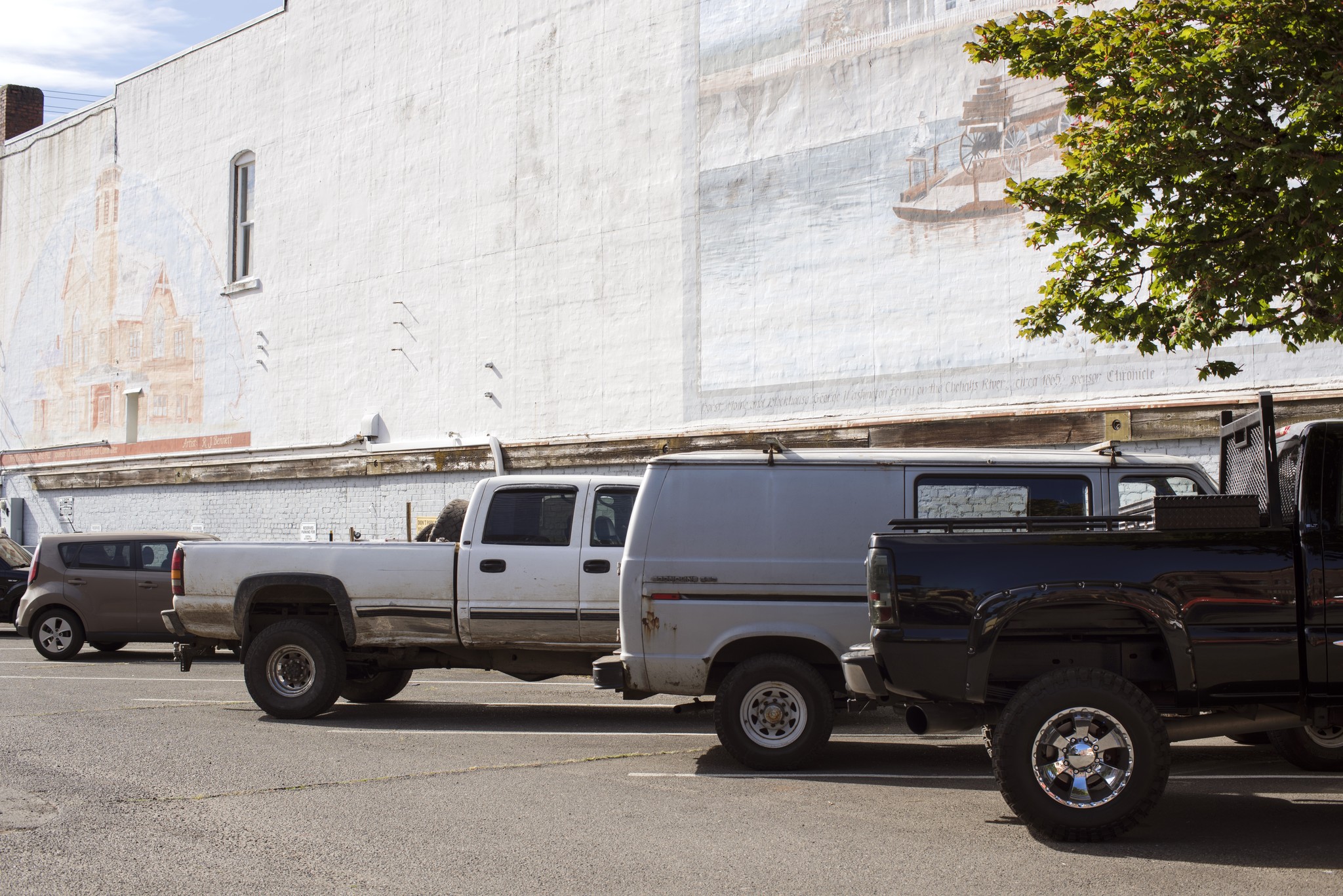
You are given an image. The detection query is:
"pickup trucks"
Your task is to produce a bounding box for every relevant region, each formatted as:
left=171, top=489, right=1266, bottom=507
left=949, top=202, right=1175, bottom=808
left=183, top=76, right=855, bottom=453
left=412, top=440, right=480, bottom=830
left=847, top=394, right=1343, bottom=846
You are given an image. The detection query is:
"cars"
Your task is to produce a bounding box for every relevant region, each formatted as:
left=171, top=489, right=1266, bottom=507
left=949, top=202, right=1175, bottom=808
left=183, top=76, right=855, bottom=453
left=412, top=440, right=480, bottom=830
left=0, top=534, right=34, bottom=627
left=161, top=476, right=643, bottom=720
left=15, top=532, right=240, bottom=662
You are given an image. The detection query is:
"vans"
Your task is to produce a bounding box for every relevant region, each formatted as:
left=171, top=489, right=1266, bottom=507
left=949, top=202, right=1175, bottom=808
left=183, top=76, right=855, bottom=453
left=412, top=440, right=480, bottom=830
left=590, top=435, right=1230, bottom=771
